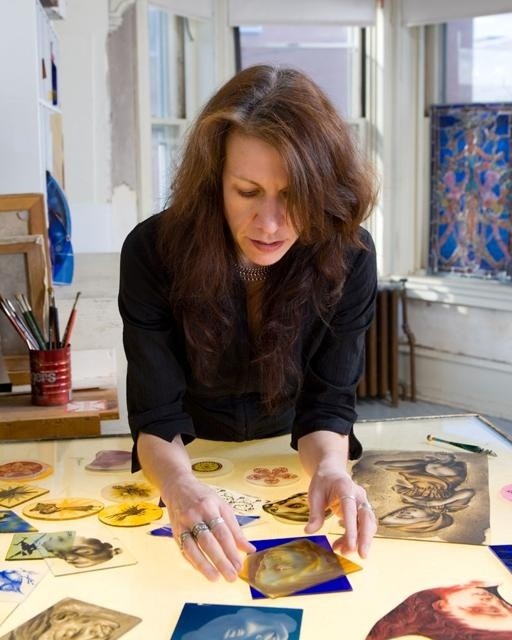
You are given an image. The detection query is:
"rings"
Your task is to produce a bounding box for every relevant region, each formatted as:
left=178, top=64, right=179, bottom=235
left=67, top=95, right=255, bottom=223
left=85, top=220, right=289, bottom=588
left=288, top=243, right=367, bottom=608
left=357, top=503, right=371, bottom=509
left=338, top=494, right=355, bottom=502
left=173, top=530, right=193, bottom=553
left=209, top=518, right=225, bottom=528
left=189, top=522, right=207, bottom=540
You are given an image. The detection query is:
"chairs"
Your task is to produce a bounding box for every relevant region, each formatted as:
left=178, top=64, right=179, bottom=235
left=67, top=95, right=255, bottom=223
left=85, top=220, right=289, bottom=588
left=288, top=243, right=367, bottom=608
left=0, top=412, right=103, bottom=442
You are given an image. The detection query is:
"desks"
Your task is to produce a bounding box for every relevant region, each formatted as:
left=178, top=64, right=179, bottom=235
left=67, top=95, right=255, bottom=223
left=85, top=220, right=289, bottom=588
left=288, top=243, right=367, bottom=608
left=1, top=403, right=510, bottom=640
left=0, top=383, right=121, bottom=423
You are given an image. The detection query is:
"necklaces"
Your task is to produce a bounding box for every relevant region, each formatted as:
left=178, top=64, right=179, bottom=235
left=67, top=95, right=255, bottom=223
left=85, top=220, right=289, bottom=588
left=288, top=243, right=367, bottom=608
left=231, top=263, right=267, bottom=283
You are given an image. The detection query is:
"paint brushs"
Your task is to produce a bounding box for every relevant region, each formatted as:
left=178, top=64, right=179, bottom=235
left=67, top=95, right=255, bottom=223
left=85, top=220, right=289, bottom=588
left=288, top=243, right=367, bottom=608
left=0, top=287, right=82, bottom=351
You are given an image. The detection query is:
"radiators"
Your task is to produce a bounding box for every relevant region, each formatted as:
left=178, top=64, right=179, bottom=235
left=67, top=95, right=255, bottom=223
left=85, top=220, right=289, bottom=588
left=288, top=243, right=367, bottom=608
left=352, top=279, right=419, bottom=407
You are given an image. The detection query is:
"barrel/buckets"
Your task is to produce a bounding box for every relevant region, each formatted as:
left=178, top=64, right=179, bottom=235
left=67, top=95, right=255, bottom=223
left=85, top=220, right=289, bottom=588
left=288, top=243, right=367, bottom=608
left=30, top=340, right=73, bottom=407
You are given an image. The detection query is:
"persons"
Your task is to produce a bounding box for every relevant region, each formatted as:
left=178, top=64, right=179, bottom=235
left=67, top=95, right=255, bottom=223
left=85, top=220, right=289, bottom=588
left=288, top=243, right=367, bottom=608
left=250, top=538, right=335, bottom=594
left=120, top=63, right=381, bottom=582
left=369, top=582, right=512, bottom=640
left=180, top=611, right=296, bottom=639
left=378, top=479, right=474, bottom=533
